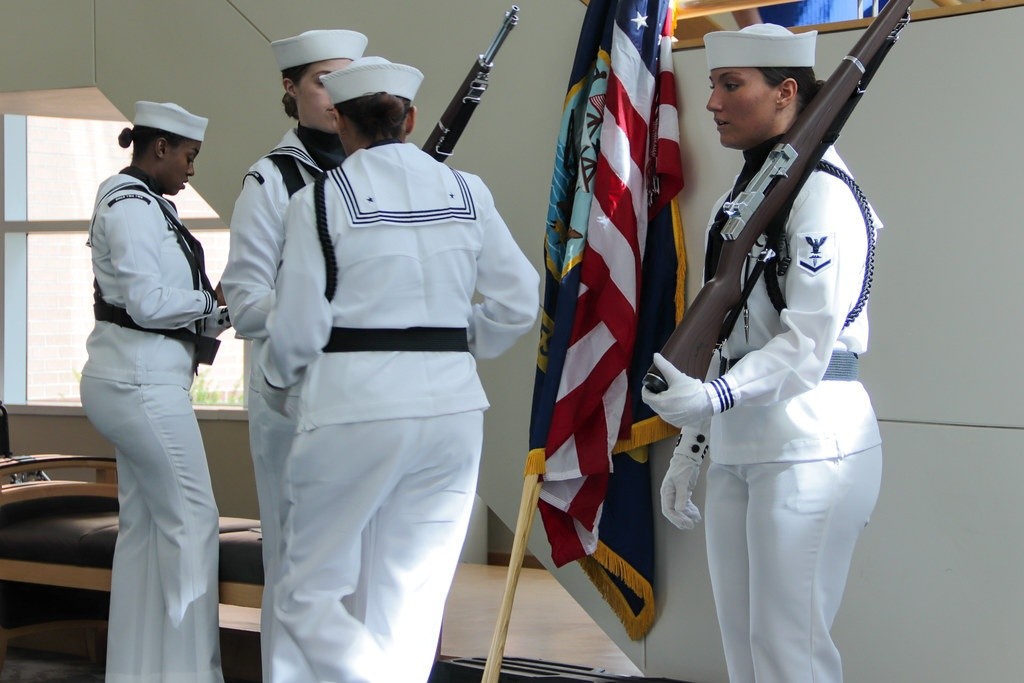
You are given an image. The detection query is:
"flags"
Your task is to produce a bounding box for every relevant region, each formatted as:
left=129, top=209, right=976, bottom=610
left=523, top=0, right=681, bottom=641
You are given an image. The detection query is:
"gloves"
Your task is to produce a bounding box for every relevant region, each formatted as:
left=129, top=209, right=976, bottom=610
left=660, top=454, right=702, bottom=531
left=259, top=374, right=293, bottom=419
left=642, top=351, right=714, bottom=428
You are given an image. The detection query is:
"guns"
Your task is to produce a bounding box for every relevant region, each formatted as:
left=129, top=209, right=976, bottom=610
left=638, top=1, right=917, bottom=397
left=418, top=4, right=522, bottom=164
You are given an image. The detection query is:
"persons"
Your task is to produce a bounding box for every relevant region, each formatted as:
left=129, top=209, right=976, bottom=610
left=259, top=56, right=540, bottom=683
left=79, top=101, right=231, bottom=683
left=641, top=22, right=883, bottom=683
left=219, top=29, right=369, bottom=683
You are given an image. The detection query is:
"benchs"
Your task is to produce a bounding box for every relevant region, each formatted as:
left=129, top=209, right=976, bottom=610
left=0, top=453, right=267, bottom=677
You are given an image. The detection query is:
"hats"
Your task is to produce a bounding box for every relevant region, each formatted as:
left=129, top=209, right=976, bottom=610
left=702, top=23, right=818, bottom=71
left=132, top=100, right=209, bottom=142
left=319, top=56, right=425, bottom=105
left=270, top=29, right=369, bottom=72
